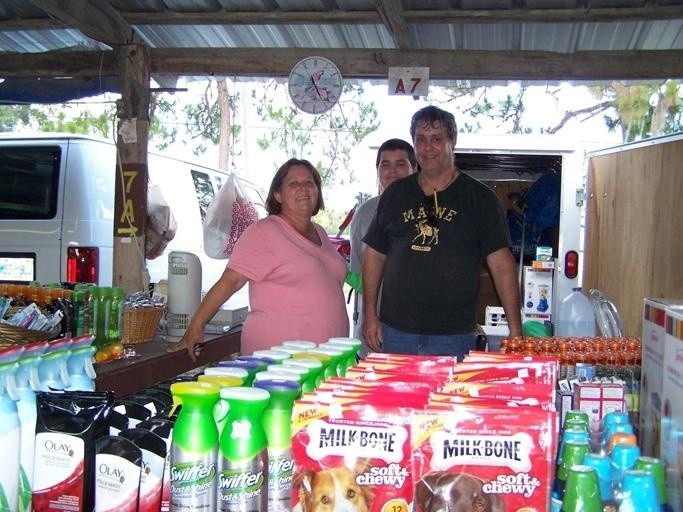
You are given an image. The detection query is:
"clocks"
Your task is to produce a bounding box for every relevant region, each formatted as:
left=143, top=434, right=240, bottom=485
left=285, top=54, right=342, bottom=117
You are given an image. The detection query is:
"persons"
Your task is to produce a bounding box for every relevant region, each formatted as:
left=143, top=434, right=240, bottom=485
left=164, top=156, right=351, bottom=365
left=346, top=135, right=420, bottom=342
left=361, top=107, right=525, bottom=361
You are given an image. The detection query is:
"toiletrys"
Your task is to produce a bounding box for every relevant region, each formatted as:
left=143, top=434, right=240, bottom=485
left=32, top=386, right=174, bottom=511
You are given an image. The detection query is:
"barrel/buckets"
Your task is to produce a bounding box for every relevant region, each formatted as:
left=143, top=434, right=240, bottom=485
left=557, top=287, right=595, bottom=338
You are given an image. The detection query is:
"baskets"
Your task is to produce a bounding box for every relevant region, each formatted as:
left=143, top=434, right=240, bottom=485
left=0, top=306, right=61, bottom=350
left=121, top=306, right=165, bottom=345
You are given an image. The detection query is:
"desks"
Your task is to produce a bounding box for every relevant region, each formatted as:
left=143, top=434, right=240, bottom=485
left=93, top=323, right=243, bottom=398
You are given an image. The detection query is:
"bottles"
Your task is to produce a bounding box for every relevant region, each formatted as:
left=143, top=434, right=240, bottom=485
left=549, top=410, right=674, bottom=512
left=499, top=335, right=642, bottom=366
left=73, top=283, right=125, bottom=348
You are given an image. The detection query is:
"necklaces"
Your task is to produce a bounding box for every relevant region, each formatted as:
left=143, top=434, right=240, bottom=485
left=422, top=166, right=457, bottom=190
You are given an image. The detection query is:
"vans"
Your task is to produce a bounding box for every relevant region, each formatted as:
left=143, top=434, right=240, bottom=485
left=0, top=130, right=268, bottom=309
left=369, top=145, right=584, bottom=337
